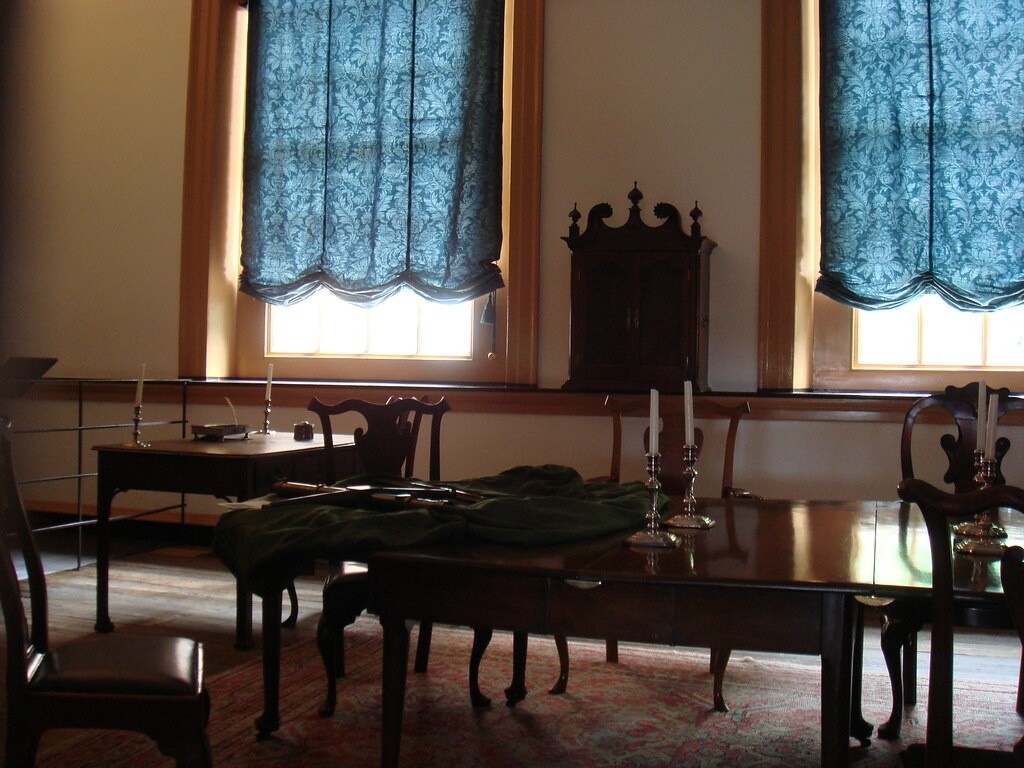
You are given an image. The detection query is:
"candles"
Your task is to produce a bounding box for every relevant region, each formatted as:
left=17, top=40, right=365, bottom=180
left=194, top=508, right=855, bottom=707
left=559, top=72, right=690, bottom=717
left=264, top=363, right=274, bottom=401
left=134, top=362, right=145, bottom=407
left=684, top=380, right=695, bottom=445
left=649, top=388, right=659, bottom=458
left=984, top=394, right=999, bottom=460
left=976, top=379, right=987, bottom=453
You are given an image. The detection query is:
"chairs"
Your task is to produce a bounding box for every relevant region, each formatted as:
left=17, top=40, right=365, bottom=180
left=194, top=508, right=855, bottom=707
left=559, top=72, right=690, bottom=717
left=278, top=396, right=455, bottom=632
left=545, top=392, right=752, bottom=713
left=0, top=420, right=213, bottom=768
left=307, top=396, right=492, bottom=721
left=876, top=379, right=1024, bottom=741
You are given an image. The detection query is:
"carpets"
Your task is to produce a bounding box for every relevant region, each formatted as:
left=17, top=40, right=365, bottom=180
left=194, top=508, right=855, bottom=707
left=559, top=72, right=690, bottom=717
left=36, top=613, right=1024, bottom=768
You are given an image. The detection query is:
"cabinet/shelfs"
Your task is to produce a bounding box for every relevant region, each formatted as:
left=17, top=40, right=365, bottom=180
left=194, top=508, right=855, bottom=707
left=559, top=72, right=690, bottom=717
left=562, top=179, right=718, bottom=395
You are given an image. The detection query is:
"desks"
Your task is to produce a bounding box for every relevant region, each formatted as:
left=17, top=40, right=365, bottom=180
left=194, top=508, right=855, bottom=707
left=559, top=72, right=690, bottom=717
left=319, top=498, right=1024, bottom=767
left=91, top=429, right=357, bottom=653
